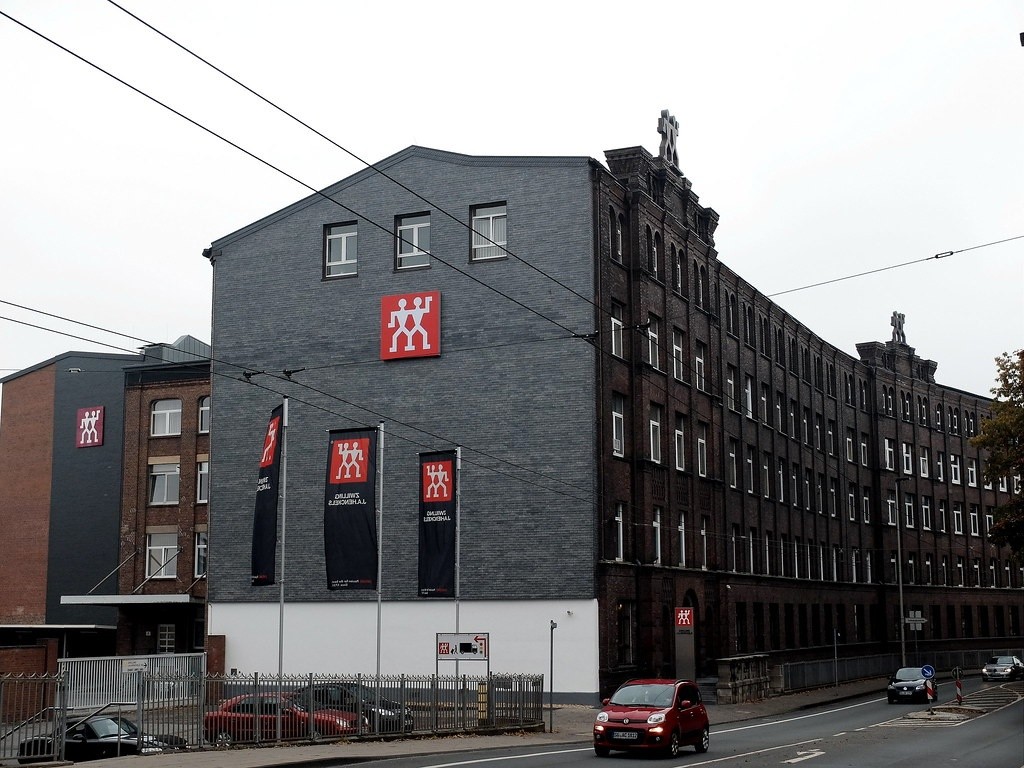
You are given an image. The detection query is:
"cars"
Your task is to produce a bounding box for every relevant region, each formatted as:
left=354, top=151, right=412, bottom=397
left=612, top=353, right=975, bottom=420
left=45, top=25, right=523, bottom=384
left=17, top=713, right=192, bottom=765
left=886, top=665, right=937, bottom=703
left=204, top=692, right=370, bottom=748
left=981, top=655, right=1024, bottom=682
left=292, top=681, right=415, bottom=736
left=593, top=677, right=710, bottom=759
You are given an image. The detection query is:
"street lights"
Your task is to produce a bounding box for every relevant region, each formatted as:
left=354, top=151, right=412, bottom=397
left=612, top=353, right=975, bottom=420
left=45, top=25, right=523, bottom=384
left=894, top=476, right=912, bottom=668
